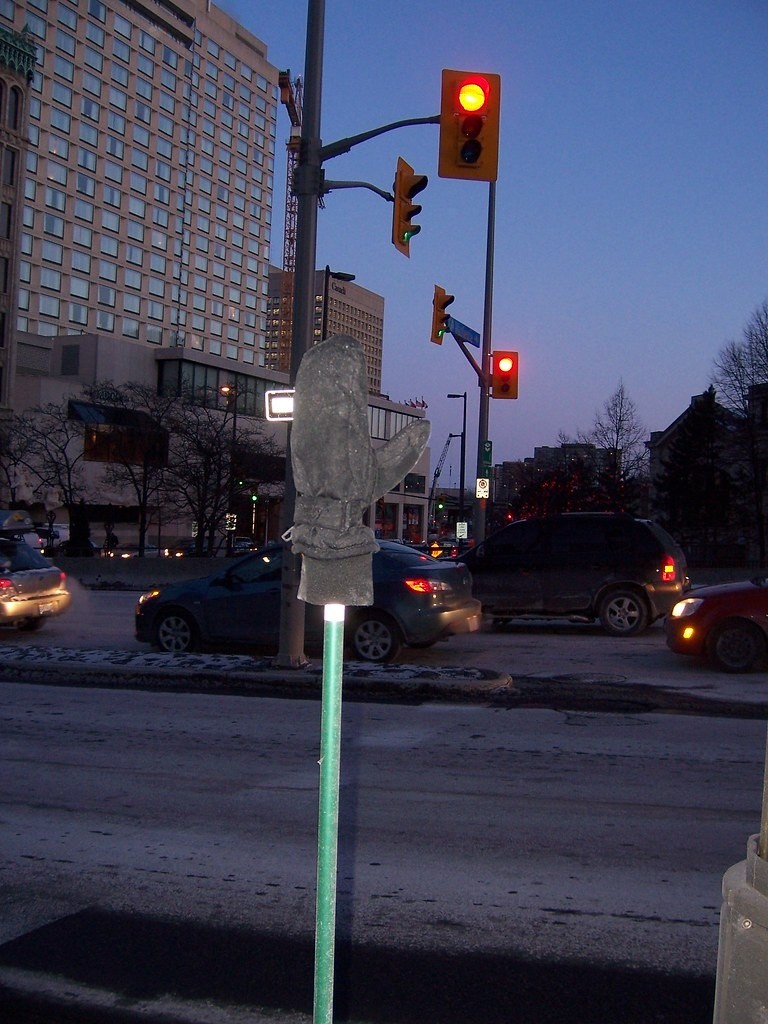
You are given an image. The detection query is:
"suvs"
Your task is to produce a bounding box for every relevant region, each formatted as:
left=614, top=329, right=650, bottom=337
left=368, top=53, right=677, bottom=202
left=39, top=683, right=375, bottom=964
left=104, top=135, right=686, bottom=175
left=436, top=511, right=690, bottom=636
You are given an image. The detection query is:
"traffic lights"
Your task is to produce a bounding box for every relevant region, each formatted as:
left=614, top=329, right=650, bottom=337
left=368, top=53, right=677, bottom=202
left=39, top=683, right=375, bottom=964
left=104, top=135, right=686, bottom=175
left=252, top=495, right=258, bottom=502
left=430, top=283, right=456, bottom=346
left=489, top=348, right=519, bottom=400
left=439, top=66, right=501, bottom=183
left=393, top=155, right=430, bottom=259
left=225, top=513, right=236, bottom=531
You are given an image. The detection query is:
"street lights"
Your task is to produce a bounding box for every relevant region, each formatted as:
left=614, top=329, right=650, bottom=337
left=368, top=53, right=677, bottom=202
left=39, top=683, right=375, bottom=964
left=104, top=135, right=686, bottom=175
left=446, top=394, right=468, bottom=522
left=219, top=384, right=237, bottom=548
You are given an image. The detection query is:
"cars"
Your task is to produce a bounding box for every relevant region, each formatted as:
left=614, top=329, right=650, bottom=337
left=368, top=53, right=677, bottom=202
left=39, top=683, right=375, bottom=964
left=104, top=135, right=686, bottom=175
left=663, top=577, right=768, bottom=674
left=112, top=542, right=169, bottom=558
left=42, top=537, right=102, bottom=558
left=0, top=539, right=69, bottom=630
left=133, top=539, right=482, bottom=665
